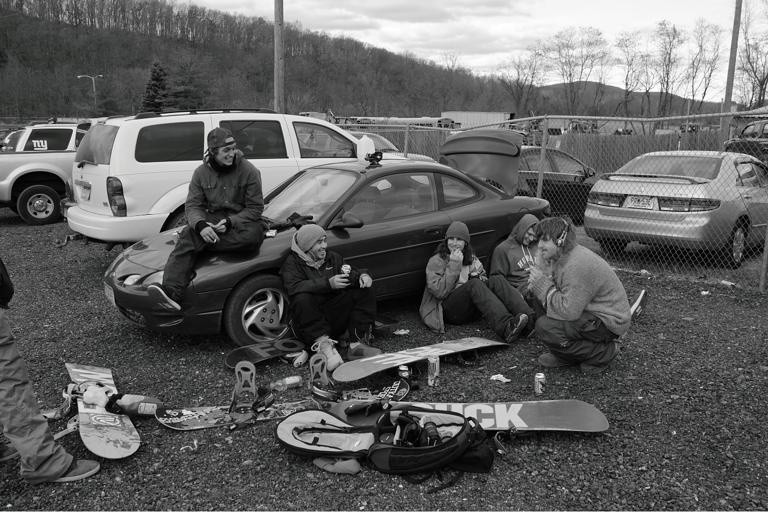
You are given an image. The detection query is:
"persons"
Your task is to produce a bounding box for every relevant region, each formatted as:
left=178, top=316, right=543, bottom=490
left=146, top=126, right=265, bottom=312
left=418, top=220, right=538, bottom=344
left=278, top=223, right=382, bottom=373
left=525, top=216, right=632, bottom=375
left=0, top=258, right=101, bottom=486
left=488, top=213, right=540, bottom=300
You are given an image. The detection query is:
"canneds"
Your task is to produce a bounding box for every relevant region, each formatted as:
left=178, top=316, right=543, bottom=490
left=427, top=355, right=440, bottom=387
left=533, top=373, right=546, bottom=396
left=340, top=264, right=350, bottom=277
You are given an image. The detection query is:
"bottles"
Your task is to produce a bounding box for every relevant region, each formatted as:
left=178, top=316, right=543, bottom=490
left=423, top=421, right=441, bottom=444
left=270, top=375, right=304, bottom=391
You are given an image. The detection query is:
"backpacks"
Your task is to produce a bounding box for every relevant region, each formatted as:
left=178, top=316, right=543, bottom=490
left=273, top=403, right=497, bottom=494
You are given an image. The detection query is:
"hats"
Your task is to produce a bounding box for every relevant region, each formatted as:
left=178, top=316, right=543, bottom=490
left=443, top=220, right=472, bottom=245
left=206, top=126, right=236, bottom=149
left=296, top=223, right=327, bottom=254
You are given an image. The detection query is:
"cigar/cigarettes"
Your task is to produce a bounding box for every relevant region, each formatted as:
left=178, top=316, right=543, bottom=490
left=214, top=239, right=217, bottom=244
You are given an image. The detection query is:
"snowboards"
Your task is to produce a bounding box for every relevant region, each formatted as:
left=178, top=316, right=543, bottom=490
left=155, top=362, right=409, bottom=430
left=224, top=315, right=400, bottom=369
left=65, top=363, right=141, bottom=459
left=332, top=336, right=511, bottom=388
left=330, top=400, right=610, bottom=435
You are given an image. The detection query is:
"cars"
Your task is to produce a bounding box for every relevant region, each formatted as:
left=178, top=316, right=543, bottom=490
left=725, top=120, right=768, bottom=166
left=675, top=123, right=698, bottom=139
left=582, top=149, right=768, bottom=270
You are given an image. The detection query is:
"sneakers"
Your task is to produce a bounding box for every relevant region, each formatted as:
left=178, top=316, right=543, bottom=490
left=579, top=344, right=620, bottom=375
left=537, top=351, right=576, bottom=368
left=0, top=440, right=19, bottom=462
left=346, top=343, right=384, bottom=361
left=46, top=457, right=101, bottom=485
left=504, top=311, right=529, bottom=343
left=628, top=287, right=649, bottom=322
left=313, top=334, right=345, bottom=372
left=146, top=282, right=183, bottom=313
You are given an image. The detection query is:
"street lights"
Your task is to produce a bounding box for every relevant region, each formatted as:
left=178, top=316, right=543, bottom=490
left=76, top=73, right=107, bottom=110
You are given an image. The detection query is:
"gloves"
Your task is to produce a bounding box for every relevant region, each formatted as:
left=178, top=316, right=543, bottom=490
left=288, top=211, right=318, bottom=231
left=341, top=396, right=394, bottom=419
